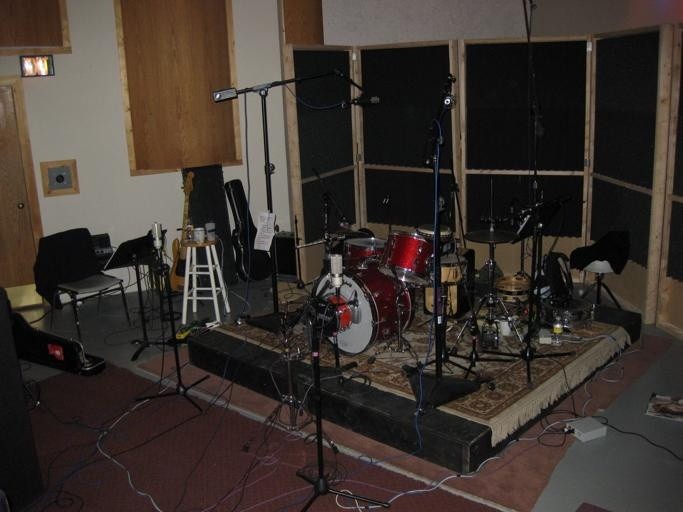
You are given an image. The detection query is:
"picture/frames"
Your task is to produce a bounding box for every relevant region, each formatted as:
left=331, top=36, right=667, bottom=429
left=20, top=54, right=55, bottom=77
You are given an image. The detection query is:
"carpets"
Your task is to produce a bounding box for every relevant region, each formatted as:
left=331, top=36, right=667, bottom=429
left=136, top=330, right=674, bottom=512
left=210, top=276, right=631, bottom=446
left=13, top=359, right=502, bottom=512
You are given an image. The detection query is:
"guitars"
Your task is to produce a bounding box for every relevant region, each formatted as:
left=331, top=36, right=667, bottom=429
left=167, top=171, right=195, bottom=292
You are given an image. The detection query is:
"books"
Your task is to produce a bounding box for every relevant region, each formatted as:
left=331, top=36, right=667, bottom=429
left=644, top=391, right=683, bottom=421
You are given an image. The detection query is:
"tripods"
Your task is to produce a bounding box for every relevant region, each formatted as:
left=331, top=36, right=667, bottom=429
left=243, top=325, right=339, bottom=456
left=108, top=269, right=211, bottom=416
left=296, top=332, right=390, bottom=512
left=410, top=156, right=578, bottom=384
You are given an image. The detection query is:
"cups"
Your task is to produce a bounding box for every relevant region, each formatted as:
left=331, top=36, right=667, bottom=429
left=204, top=223, right=215, bottom=241
left=192, top=228, right=204, bottom=243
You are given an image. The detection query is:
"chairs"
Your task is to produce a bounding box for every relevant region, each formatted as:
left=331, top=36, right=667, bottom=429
left=35, top=226, right=129, bottom=339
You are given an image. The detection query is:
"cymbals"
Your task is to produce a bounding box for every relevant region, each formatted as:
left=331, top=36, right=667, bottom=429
left=464, top=229, right=517, bottom=244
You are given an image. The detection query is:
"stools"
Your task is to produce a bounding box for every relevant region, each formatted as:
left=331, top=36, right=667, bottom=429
left=180, top=240, right=231, bottom=324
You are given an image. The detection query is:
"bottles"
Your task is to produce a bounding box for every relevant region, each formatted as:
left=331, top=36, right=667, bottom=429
left=552, top=315, right=563, bottom=347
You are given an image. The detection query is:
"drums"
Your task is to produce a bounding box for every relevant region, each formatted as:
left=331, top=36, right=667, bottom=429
left=311, top=259, right=412, bottom=356
left=346, top=238, right=387, bottom=266
left=434, top=255, right=468, bottom=284
left=385, top=234, right=430, bottom=277
left=497, top=275, right=529, bottom=303
left=417, top=221, right=453, bottom=243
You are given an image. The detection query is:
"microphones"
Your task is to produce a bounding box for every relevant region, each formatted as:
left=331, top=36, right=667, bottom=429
left=352, top=94, right=383, bottom=106
